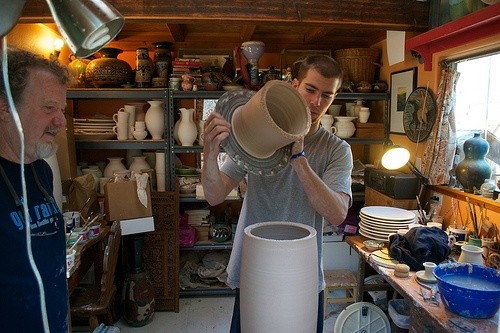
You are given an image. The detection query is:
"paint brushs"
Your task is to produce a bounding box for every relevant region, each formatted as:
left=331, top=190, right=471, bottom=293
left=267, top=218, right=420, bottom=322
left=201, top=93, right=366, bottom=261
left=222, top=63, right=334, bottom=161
left=416, top=195, right=428, bottom=225
left=81, top=215, right=99, bottom=232
left=472, top=198, right=478, bottom=234
left=81, top=217, right=90, bottom=230
left=444, top=202, right=456, bottom=219
left=79, top=196, right=93, bottom=212
left=466, top=197, right=478, bottom=238
left=452, top=198, right=459, bottom=228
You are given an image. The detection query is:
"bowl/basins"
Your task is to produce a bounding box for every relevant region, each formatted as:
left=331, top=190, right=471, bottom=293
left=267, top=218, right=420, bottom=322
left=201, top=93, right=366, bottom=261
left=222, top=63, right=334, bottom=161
left=434, top=263, right=500, bottom=319
left=387, top=298, right=410, bottom=328
left=175, top=166, right=200, bottom=194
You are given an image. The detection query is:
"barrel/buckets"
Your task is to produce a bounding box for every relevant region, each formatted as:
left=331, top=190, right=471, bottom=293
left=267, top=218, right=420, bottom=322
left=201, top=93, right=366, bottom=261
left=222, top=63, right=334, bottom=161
left=62, top=211, right=101, bottom=270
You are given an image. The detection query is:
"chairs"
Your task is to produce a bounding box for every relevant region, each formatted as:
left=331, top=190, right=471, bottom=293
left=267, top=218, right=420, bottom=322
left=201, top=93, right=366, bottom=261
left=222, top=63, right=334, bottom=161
left=70, top=220, right=121, bottom=331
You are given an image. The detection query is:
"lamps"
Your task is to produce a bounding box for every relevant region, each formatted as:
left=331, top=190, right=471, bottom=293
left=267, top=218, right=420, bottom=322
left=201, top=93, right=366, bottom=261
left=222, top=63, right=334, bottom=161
left=381, top=139, right=428, bottom=225
left=68, top=40, right=172, bottom=88
left=46, top=0, right=125, bottom=59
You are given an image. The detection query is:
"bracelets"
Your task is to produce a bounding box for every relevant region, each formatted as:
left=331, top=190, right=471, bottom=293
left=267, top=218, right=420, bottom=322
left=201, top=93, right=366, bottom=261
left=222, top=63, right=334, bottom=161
left=290, top=151, right=306, bottom=162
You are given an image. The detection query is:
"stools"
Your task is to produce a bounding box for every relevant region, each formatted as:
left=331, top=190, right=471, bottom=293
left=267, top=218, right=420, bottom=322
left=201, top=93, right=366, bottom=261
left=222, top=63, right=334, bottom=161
left=323, top=269, right=357, bottom=320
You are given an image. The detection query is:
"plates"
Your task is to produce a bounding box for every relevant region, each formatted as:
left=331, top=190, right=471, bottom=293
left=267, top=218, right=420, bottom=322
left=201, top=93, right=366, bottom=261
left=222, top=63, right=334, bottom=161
left=358, top=205, right=418, bottom=243
left=73, top=116, right=116, bottom=139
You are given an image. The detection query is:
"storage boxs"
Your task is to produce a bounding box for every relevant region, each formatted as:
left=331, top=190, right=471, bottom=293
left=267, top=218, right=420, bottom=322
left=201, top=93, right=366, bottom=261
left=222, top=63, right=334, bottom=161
left=104, top=180, right=155, bottom=235
left=281, top=48, right=331, bottom=70
left=364, top=167, right=420, bottom=210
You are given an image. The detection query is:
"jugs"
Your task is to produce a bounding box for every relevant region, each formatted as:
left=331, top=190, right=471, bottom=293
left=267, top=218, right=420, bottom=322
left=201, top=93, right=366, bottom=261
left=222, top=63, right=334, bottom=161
left=182, top=75, right=195, bottom=92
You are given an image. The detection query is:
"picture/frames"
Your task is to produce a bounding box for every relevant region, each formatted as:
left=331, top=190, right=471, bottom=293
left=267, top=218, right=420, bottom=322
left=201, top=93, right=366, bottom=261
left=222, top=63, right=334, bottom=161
left=389, top=67, right=417, bottom=135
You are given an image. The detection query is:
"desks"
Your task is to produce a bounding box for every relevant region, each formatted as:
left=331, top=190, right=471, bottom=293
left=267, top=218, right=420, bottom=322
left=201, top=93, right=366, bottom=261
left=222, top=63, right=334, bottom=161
left=345, top=236, right=500, bottom=333
left=67, top=226, right=109, bottom=333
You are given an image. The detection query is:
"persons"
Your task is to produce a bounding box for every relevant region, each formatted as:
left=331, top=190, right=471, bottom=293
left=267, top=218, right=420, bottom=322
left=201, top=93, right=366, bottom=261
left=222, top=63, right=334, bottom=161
left=1, top=50, right=72, bottom=333
left=202, top=54, right=353, bottom=333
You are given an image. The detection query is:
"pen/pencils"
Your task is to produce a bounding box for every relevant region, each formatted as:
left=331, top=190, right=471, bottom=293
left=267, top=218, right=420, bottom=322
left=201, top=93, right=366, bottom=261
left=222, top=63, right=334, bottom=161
left=71, top=212, right=74, bottom=219
left=66, top=235, right=83, bottom=254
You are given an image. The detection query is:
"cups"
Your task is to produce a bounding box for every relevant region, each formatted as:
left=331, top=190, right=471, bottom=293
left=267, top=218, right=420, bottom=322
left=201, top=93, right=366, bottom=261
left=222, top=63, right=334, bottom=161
left=113, top=103, right=135, bottom=140
left=449, top=225, right=494, bottom=250
left=417, top=222, right=443, bottom=231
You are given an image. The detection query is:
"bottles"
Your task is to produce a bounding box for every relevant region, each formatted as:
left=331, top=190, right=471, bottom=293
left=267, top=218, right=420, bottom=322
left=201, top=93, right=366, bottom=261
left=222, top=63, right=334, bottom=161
left=457, top=243, right=484, bottom=266
left=77, top=152, right=166, bottom=195
left=132, top=101, right=206, bottom=150
left=321, top=101, right=371, bottom=140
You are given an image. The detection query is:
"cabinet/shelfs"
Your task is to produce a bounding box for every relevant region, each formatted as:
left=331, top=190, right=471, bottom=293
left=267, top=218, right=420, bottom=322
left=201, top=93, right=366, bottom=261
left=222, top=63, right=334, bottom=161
left=66, top=89, right=170, bottom=193
left=169, top=88, right=388, bottom=296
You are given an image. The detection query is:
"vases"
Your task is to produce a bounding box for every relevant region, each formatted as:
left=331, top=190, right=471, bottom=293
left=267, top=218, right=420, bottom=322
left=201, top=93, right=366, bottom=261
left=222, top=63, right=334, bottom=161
left=76, top=151, right=165, bottom=192
left=128, top=101, right=164, bottom=141
left=174, top=108, right=198, bottom=146
